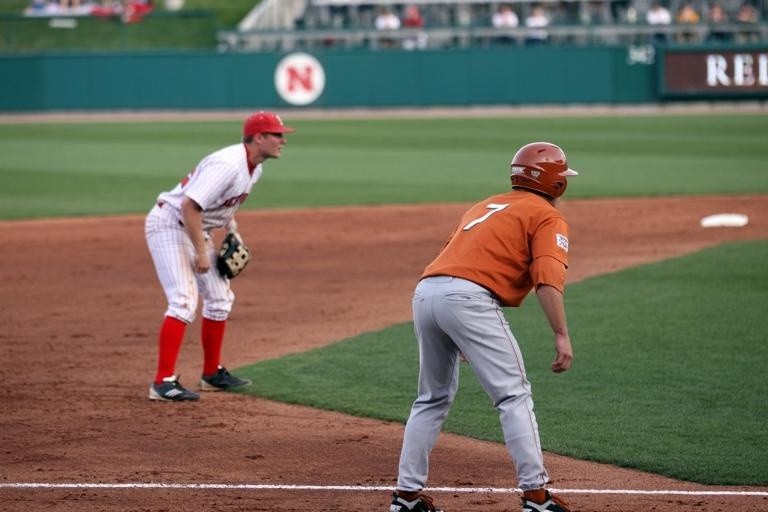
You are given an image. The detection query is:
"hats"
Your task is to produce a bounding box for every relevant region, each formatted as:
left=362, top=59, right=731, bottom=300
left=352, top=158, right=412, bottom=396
left=244, top=113, right=292, bottom=137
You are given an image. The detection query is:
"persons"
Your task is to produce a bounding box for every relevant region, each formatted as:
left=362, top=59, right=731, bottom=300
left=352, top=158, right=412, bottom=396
left=386, top=140, right=573, bottom=512
left=144, top=112, right=296, bottom=402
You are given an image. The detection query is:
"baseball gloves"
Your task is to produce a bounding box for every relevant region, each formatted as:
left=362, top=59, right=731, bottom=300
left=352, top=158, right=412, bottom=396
left=215, top=228, right=249, bottom=275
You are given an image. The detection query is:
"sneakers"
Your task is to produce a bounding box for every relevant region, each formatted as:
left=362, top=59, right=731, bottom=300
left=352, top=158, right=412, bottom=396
left=389, top=491, right=443, bottom=512
left=148, top=374, right=200, bottom=401
left=521, top=489, right=572, bottom=512
left=200, top=365, right=252, bottom=391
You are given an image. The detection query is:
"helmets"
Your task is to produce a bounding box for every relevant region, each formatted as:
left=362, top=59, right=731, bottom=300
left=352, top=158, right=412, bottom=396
left=510, top=141, right=578, bottom=198
